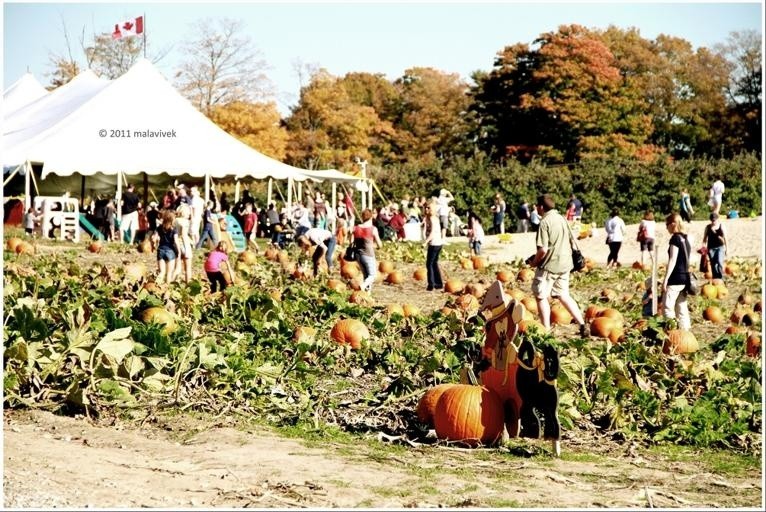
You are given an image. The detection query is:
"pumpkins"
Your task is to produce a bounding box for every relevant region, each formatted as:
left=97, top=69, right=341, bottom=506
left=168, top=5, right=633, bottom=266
left=416, top=384, right=461, bottom=429
left=142, top=308, right=175, bottom=338
left=442, top=257, right=761, bottom=356
left=223, top=248, right=429, bottom=318
left=434, top=384, right=504, bottom=447
left=6, top=237, right=34, bottom=256
left=330, top=319, right=371, bottom=352
left=88, top=244, right=97, bottom=252
left=139, top=238, right=152, bottom=253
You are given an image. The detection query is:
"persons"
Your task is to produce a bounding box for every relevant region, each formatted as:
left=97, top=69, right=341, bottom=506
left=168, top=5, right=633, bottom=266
left=678, top=188, right=695, bottom=223
left=661, top=212, right=692, bottom=330
left=353, top=188, right=506, bottom=292
left=638, top=213, right=657, bottom=270
left=707, top=174, right=725, bottom=214
left=703, top=213, right=728, bottom=279
left=605, top=209, right=626, bottom=268
left=25, top=181, right=355, bottom=298
left=515, top=193, right=584, bottom=233
left=641, top=277, right=664, bottom=317
left=530, top=192, right=592, bottom=339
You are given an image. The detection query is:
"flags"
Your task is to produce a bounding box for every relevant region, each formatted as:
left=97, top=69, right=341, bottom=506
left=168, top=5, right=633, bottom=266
left=112, top=15, right=143, bottom=39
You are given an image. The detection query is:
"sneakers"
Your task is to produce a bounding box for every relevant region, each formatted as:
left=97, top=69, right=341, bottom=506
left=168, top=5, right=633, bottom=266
left=580, top=324, right=592, bottom=339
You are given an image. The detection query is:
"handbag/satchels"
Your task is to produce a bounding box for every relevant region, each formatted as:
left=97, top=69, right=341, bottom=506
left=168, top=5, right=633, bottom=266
left=342, top=244, right=358, bottom=262
left=570, top=249, right=586, bottom=273
left=636, top=230, right=649, bottom=242
left=685, top=271, right=700, bottom=296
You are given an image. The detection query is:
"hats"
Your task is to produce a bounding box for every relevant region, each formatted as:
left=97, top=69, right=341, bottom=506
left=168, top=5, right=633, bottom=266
left=642, top=277, right=664, bottom=292
